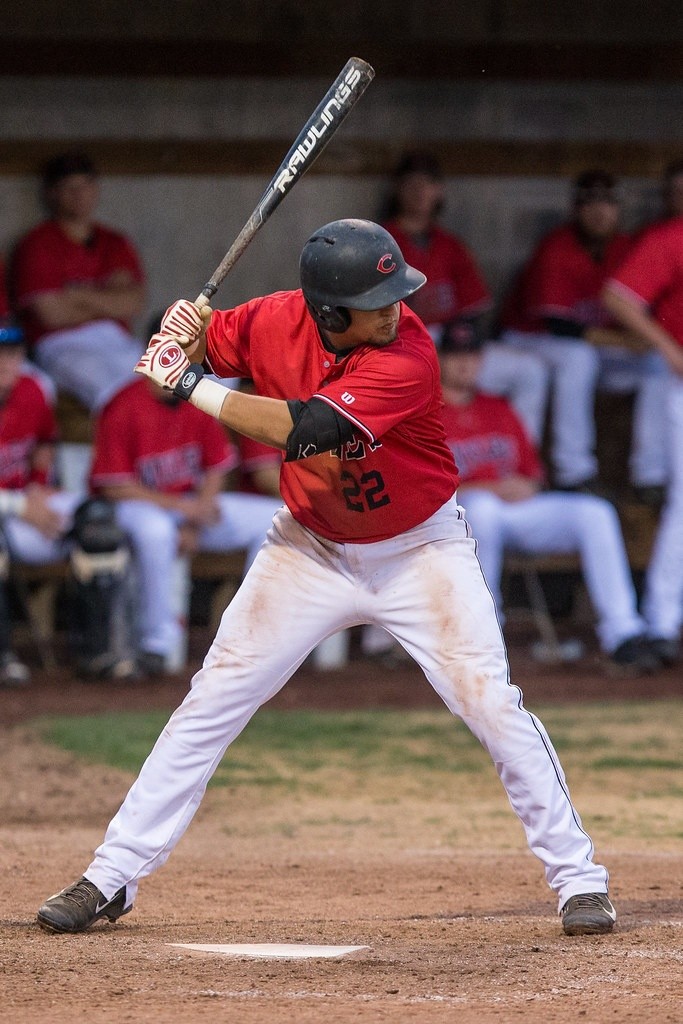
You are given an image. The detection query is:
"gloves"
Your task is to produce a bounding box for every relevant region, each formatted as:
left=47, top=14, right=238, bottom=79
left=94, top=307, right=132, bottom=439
left=133, top=333, right=204, bottom=401
left=160, top=299, right=213, bottom=359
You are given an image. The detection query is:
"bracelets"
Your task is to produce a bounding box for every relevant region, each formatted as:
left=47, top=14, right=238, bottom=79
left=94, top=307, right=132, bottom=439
left=188, top=378, right=231, bottom=419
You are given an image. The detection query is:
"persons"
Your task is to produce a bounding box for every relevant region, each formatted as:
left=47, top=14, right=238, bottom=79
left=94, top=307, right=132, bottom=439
left=86, top=311, right=346, bottom=679
left=37, top=216, right=617, bottom=937
left=0, top=327, right=135, bottom=677
left=240, top=386, right=414, bottom=671
left=425, top=317, right=648, bottom=672
left=370, top=150, right=598, bottom=498
left=15, top=163, right=151, bottom=411
left=525, top=170, right=679, bottom=498
left=606, top=204, right=683, bottom=672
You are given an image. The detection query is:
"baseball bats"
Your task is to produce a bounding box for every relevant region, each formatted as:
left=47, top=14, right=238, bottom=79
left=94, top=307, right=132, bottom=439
left=193, top=56, right=375, bottom=308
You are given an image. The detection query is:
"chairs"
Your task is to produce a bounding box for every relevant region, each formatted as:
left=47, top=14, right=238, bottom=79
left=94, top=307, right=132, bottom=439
left=0, top=517, right=682, bottom=670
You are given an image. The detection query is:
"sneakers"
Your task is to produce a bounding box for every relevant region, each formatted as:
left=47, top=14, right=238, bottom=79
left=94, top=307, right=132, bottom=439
left=36, top=875, right=133, bottom=935
left=562, top=892, right=617, bottom=934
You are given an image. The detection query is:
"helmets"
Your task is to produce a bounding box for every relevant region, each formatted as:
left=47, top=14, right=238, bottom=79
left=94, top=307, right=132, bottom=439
left=299, top=219, right=426, bottom=333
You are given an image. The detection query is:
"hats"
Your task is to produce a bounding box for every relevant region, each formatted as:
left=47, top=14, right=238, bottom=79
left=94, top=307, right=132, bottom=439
left=436, top=319, right=484, bottom=358
